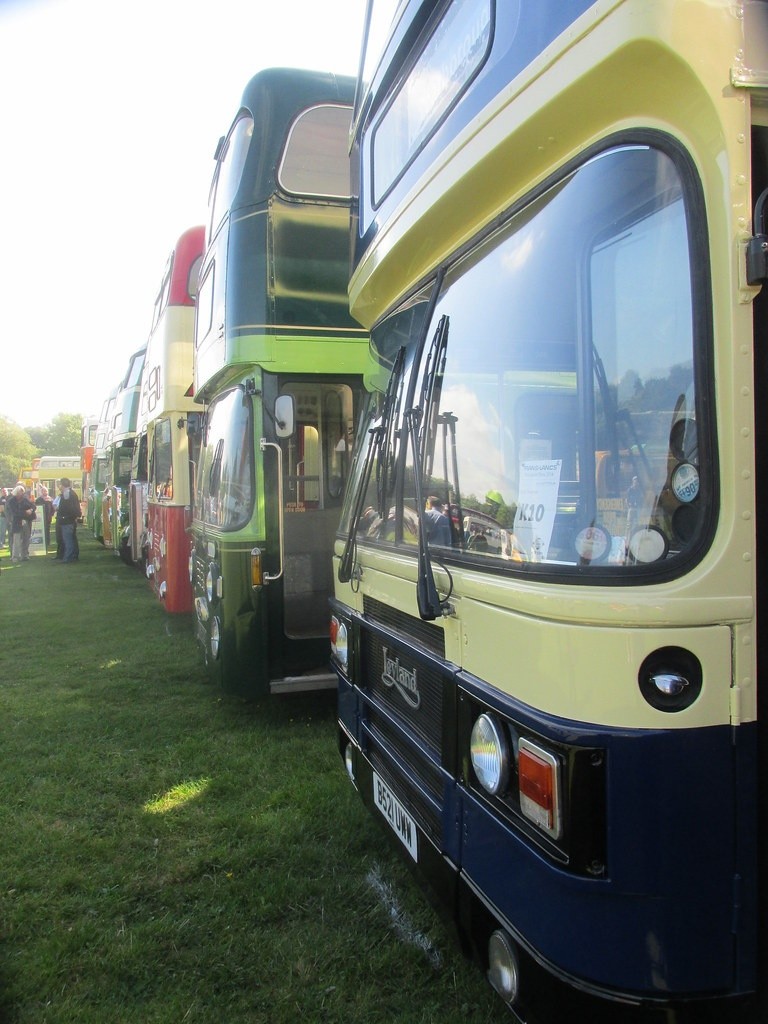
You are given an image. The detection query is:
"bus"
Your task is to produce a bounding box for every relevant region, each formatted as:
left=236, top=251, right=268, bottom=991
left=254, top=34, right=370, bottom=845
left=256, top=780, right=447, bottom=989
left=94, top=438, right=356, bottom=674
left=85, top=221, right=202, bottom=619
left=328, top=0, right=768, bottom=1024
left=17, top=456, right=83, bottom=506
left=187, top=66, right=572, bottom=693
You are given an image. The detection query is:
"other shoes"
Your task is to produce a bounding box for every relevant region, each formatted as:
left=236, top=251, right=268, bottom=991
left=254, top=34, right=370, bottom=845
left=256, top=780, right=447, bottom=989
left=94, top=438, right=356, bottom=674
left=58, top=560, right=76, bottom=564
left=12, top=557, right=32, bottom=561
left=50, top=557, right=59, bottom=560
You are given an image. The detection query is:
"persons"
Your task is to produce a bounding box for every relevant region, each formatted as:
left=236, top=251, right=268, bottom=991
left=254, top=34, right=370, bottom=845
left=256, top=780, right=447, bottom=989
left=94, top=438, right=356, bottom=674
left=0, top=476, right=83, bottom=566
left=419, top=495, right=489, bottom=553
